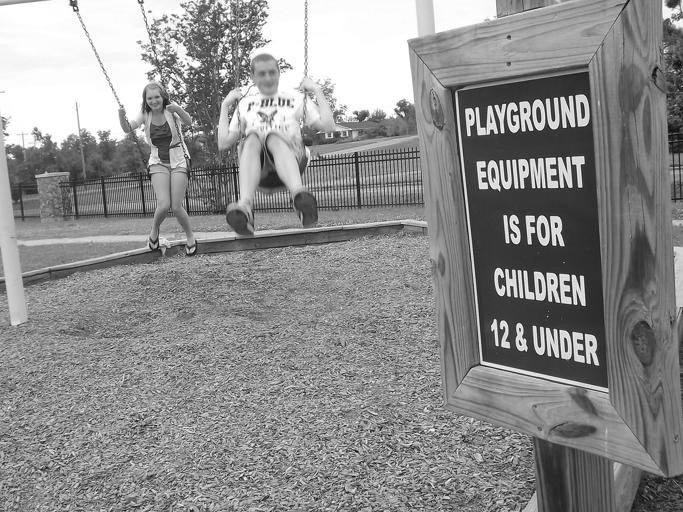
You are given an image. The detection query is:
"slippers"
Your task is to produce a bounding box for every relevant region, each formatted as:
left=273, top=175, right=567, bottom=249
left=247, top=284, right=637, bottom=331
left=185, top=239, right=197, bottom=256
left=149, top=226, right=160, bottom=252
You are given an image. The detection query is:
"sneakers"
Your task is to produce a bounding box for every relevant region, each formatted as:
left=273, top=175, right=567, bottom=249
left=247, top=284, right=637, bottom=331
left=289, top=188, right=318, bottom=226
left=225, top=199, right=255, bottom=236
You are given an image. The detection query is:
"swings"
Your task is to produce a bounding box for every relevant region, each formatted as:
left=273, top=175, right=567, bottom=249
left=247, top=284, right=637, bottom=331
left=70, top=0, right=190, bottom=182
left=235, top=0, right=308, bottom=188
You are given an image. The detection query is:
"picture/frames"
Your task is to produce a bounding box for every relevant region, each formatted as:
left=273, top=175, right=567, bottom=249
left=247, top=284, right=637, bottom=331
left=406, top=0, right=683, bottom=483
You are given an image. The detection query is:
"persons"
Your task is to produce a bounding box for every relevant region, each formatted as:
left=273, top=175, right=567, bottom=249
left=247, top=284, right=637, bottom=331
left=216, top=54, right=336, bottom=236
left=114, top=82, right=197, bottom=256
